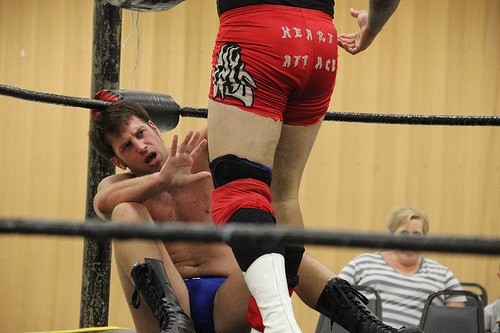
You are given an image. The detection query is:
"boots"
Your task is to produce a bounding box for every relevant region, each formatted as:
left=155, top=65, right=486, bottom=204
left=129, top=257, right=194, bottom=332
left=316, top=277, right=422, bottom=332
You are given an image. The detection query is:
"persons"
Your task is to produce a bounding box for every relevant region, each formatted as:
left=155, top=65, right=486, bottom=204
left=337, top=207, right=467, bottom=330
left=483, top=263, right=500, bottom=333
left=205, top=0, right=400, bottom=333
left=90, top=100, right=425, bottom=333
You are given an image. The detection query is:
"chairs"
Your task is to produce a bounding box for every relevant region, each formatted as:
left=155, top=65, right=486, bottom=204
left=314, top=285, right=382, bottom=333
left=418, top=281, right=488, bottom=333
